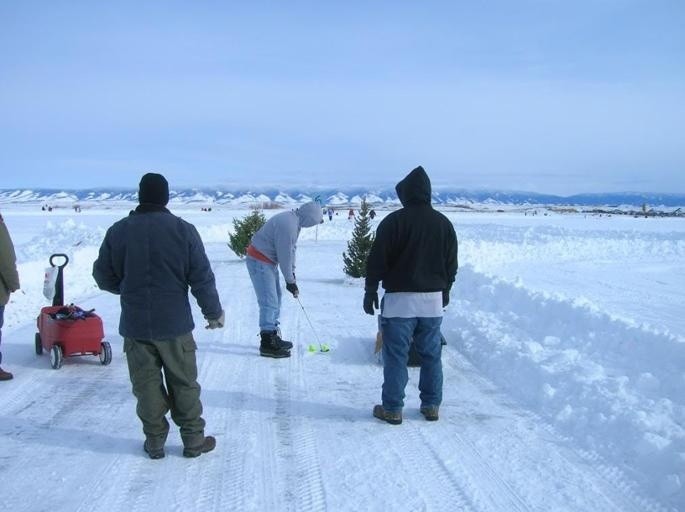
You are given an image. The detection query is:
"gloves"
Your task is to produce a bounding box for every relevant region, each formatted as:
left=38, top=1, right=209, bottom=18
left=207, top=309, right=226, bottom=328
left=286, top=283, right=299, bottom=295
left=363, top=292, right=378, bottom=315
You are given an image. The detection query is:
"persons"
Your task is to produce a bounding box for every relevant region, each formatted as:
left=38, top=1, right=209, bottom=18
left=348, top=208, right=355, bottom=220
left=92, top=172, right=226, bottom=460
left=0, top=215, right=22, bottom=383
left=326, top=207, right=335, bottom=221
left=244, top=199, right=325, bottom=360
left=359, top=164, right=458, bottom=425
left=369, top=209, right=376, bottom=219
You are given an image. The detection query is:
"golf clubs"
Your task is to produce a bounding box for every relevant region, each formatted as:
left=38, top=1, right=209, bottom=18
left=296, top=296, right=331, bottom=352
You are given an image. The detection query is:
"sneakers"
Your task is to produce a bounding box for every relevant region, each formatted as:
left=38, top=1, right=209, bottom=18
left=183, top=437, right=216, bottom=457
left=421, top=408, right=439, bottom=420
left=144, top=441, right=165, bottom=458
left=374, top=405, right=402, bottom=424
left=260, top=330, right=292, bottom=357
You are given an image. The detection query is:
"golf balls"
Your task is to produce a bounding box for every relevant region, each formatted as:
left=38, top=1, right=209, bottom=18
left=309, top=344, right=329, bottom=351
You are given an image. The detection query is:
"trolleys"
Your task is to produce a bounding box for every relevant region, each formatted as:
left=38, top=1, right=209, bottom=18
left=34, top=253, right=111, bottom=369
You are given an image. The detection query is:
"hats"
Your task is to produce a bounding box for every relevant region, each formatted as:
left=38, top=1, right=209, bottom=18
left=138, top=174, right=168, bottom=205
